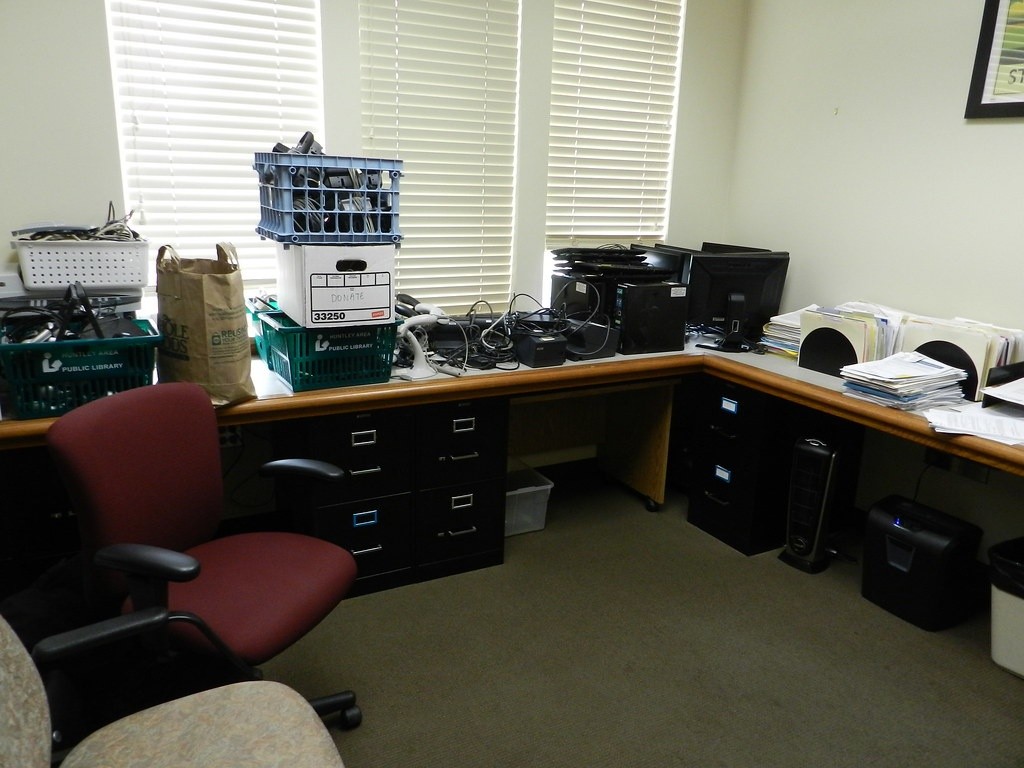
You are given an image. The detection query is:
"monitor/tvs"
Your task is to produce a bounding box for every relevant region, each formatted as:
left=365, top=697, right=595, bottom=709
left=630, top=241, right=790, bottom=353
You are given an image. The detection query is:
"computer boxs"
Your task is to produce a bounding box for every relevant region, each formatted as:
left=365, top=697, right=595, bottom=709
left=550, top=274, right=688, bottom=355
left=776, top=433, right=863, bottom=575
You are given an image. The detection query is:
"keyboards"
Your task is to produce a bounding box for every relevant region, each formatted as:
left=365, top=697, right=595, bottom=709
left=551, top=248, right=677, bottom=282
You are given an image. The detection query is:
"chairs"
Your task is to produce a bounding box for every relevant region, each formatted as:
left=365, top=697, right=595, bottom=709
left=47, top=382, right=361, bottom=731
left=0, top=609, right=346, bottom=768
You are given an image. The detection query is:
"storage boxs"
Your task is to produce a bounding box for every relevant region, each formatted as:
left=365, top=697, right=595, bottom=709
left=255, top=152, right=404, bottom=245
left=277, top=242, right=396, bottom=328
left=505, top=455, right=554, bottom=535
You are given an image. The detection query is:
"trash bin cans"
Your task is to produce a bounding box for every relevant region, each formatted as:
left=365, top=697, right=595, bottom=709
left=989, top=535, right=1024, bottom=680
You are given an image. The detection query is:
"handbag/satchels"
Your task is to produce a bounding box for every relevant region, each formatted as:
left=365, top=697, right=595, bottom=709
left=156, top=241, right=258, bottom=411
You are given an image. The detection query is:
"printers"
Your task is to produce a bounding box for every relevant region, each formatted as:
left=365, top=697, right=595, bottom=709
left=860, top=493, right=991, bottom=633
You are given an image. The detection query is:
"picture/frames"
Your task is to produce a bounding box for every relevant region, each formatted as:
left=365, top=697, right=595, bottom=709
left=964, top=0, right=1024, bottom=118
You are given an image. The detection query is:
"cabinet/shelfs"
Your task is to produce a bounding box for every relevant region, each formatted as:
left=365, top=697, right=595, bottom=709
left=275, top=395, right=510, bottom=600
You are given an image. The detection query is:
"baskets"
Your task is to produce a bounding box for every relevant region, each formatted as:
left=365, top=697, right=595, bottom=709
left=0, top=318, right=165, bottom=418
left=245, top=295, right=405, bottom=392
left=10, top=234, right=149, bottom=291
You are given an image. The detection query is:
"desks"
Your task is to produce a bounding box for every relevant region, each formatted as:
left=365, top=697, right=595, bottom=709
left=0, top=353, right=1024, bottom=513
